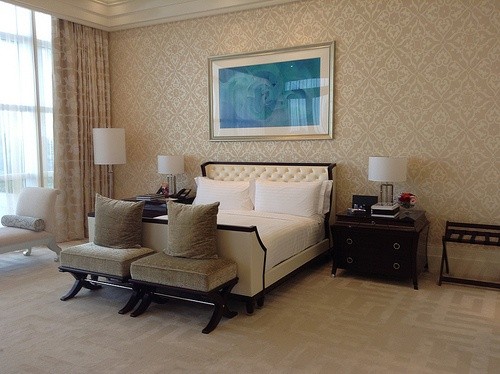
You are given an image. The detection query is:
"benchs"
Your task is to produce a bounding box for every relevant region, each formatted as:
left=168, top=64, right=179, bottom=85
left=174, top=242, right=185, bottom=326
left=57, top=242, right=240, bottom=334
left=0, top=186, right=63, bottom=263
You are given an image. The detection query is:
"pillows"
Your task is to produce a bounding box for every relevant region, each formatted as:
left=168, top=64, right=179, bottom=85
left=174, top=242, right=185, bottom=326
left=162, top=199, right=220, bottom=259
left=1, top=215, right=45, bottom=231
left=192, top=177, right=333, bottom=224
left=94, top=193, right=145, bottom=249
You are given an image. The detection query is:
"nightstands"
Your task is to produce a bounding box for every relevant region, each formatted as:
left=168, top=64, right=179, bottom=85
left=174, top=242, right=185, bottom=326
left=329, top=210, right=431, bottom=291
left=124, top=193, right=196, bottom=218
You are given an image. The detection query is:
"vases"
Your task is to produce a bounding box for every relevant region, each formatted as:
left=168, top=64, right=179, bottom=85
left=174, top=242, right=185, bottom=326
left=399, top=204, right=417, bottom=221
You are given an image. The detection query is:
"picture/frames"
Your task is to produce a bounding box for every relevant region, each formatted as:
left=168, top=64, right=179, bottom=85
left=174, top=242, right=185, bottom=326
left=208, top=40, right=336, bottom=142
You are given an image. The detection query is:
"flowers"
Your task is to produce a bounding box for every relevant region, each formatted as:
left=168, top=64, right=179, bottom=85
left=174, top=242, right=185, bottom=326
left=398, top=192, right=417, bottom=209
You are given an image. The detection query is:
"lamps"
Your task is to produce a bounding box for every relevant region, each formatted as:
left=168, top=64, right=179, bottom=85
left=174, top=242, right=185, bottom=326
left=368, top=157, right=409, bottom=220
left=93, top=128, right=125, bottom=198
left=158, top=156, right=184, bottom=195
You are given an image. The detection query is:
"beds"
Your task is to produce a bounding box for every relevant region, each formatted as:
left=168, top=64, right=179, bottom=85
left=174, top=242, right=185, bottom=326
left=88, top=162, right=337, bottom=314
